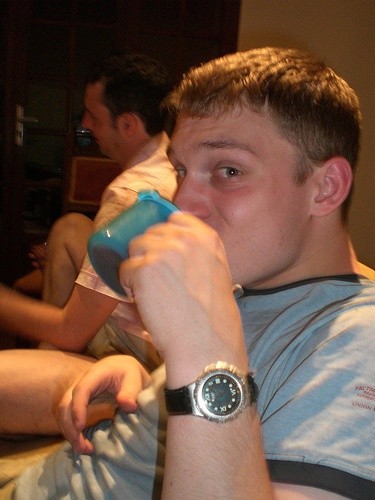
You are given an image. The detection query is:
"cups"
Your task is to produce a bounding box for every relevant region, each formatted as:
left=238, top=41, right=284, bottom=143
left=87, top=189, right=178, bottom=296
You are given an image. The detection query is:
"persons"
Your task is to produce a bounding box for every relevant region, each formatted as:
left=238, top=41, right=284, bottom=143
left=1, top=63, right=181, bottom=381
left=0, top=48, right=375, bottom=500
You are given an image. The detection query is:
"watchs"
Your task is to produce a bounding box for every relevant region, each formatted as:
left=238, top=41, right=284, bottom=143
left=165, top=364, right=261, bottom=423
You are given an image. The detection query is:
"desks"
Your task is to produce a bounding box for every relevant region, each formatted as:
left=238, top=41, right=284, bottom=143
left=63, top=153, right=123, bottom=217
